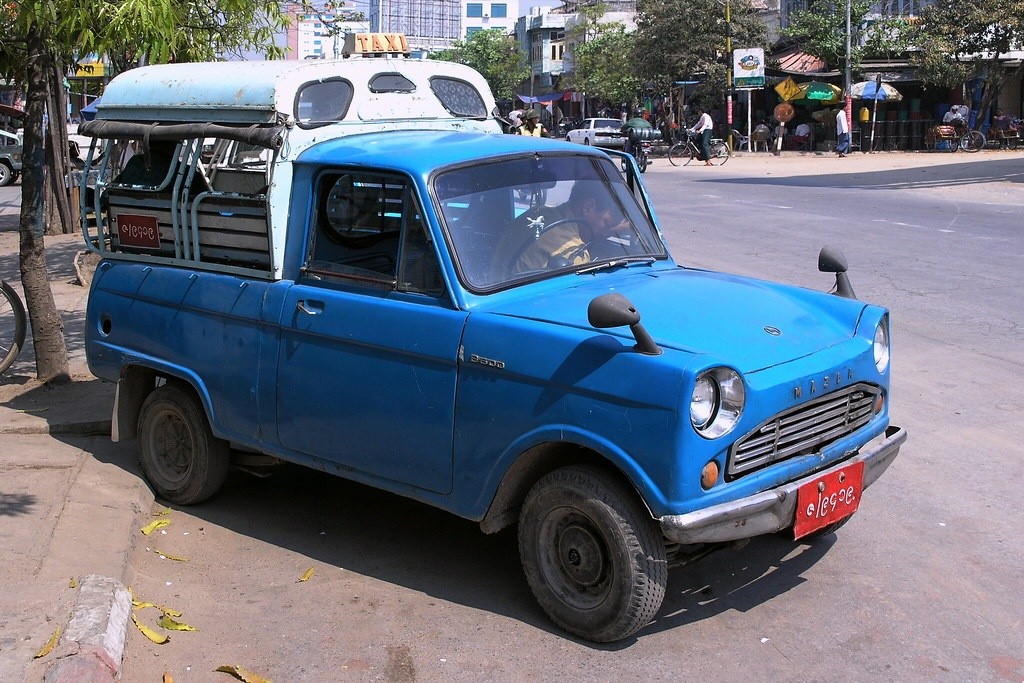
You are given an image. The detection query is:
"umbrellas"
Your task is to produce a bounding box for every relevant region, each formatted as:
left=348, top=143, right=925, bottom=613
left=508, top=108, right=527, bottom=120
left=844, top=80, right=904, bottom=103
left=778, top=79, right=842, bottom=106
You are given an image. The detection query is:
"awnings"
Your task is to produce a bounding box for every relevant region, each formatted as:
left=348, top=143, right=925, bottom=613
left=514, top=92, right=564, bottom=103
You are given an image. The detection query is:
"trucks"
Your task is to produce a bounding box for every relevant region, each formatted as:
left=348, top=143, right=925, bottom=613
left=79, top=57, right=908, bottom=642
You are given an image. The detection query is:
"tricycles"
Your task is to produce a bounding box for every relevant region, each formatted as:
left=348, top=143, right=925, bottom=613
left=925, top=119, right=985, bottom=153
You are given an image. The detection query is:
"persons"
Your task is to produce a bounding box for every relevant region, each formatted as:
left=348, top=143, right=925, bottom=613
left=943, top=105, right=966, bottom=125
left=518, top=109, right=544, bottom=137
left=513, top=114, right=521, bottom=127
left=745, top=116, right=811, bottom=152
left=489, top=178, right=631, bottom=284
left=515, top=116, right=527, bottom=135
left=688, top=107, right=714, bottom=166
left=621, top=109, right=652, bottom=163
left=992, top=109, right=1013, bottom=151
left=836, top=103, right=849, bottom=157
left=672, top=120, right=680, bottom=138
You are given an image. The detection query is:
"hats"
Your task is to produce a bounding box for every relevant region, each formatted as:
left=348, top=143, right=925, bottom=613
left=525, top=109, right=540, bottom=119
left=951, top=105, right=958, bottom=110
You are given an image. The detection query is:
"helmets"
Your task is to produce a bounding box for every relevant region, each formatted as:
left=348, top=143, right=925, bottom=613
left=633, top=109, right=644, bottom=117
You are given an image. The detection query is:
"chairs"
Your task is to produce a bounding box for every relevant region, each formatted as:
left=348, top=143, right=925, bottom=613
left=732, top=129, right=754, bottom=151
left=753, top=129, right=769, bottom=153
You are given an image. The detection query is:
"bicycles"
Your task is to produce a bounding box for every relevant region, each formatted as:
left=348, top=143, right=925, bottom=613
left=0, top=279, right=27, bottom=375
left=620, top=130, right=651, bottom=172
left=668, top=125, right=730, bottom=166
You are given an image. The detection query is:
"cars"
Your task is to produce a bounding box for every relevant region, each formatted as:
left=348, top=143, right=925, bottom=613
left=565, top=118, right=629, bottom=148
left=0, top=105, right=24, bottom=186
